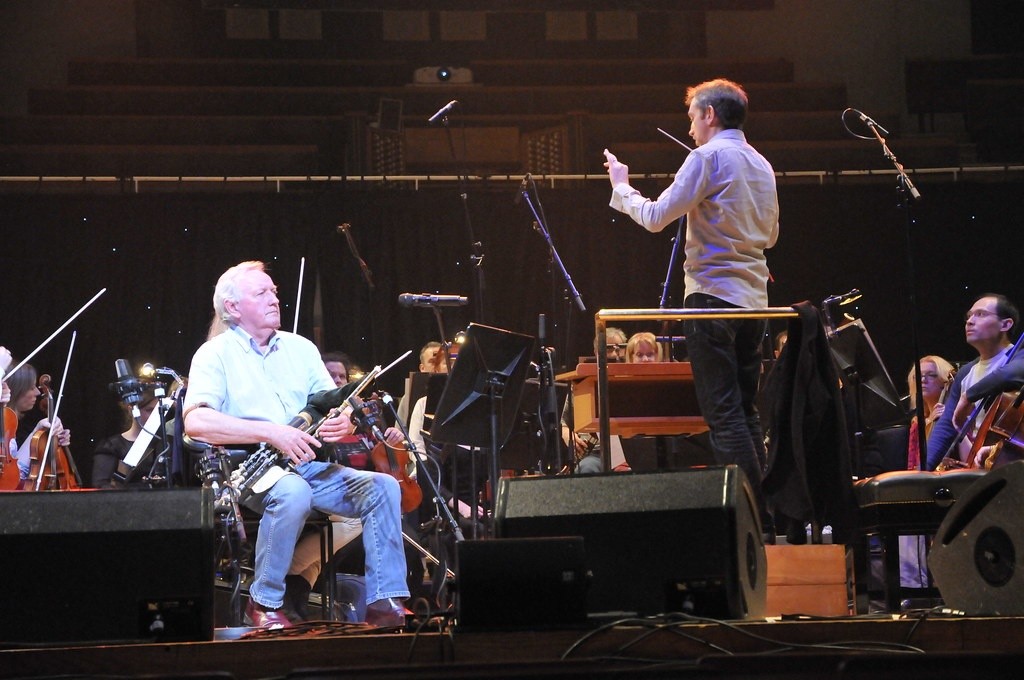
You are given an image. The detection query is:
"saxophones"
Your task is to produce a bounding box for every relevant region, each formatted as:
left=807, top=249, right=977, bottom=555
left=215, top=363, right=384, bottom=514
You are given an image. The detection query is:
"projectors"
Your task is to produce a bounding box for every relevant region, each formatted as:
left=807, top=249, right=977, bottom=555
left=414, top=65, right=474, bottom=83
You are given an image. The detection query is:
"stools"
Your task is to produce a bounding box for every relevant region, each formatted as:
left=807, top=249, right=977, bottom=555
left=851, top=469, right=991, bottom=612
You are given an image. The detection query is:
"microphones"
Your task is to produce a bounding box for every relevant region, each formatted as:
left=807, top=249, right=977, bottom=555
left=202, top=448, right=223, bottom=501
left=852, top=108, right=889, bottom=137
left=398, top=291, right=468, bottom=307
left=348, top=396, right=386, bottom=442
left=428, top=100, right=457, bottom=126
left=515, top=174, right=529, bottom=204
left=116, top=359, right=141, bottom=421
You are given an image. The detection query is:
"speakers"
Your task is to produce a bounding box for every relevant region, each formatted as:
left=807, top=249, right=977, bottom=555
left=454, top=536, right=590, bottom=633
left=324, top=574, right=369, bottom=623
left=0, top=487, right=218, bottom=651
left=928, top=459, right=1024, bottom=617
left=493, top=463, right=767, bottom=624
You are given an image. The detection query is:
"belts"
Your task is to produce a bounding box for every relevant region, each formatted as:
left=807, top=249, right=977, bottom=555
left=223, top=443, right=264, bottom=456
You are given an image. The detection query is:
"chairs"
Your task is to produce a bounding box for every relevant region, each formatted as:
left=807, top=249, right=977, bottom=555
left=175, top=386, right=338, bottom=626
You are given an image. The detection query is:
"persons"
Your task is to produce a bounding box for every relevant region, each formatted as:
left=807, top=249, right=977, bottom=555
left=603, top=78, right=780, bottom=547
left=0, top=346, right=70, bottom=490
left=183, top=261, right=447, bottom=631
left=775, top=330, right=788, bottom=359
left=91, top=341, right=483, bottom=630
left=561, top=327, right=663, bottom=475
left=852, top=293, right=1024, bottom=614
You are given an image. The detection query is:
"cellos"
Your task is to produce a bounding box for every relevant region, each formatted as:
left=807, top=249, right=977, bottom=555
left=934, top=333, right=1023, bottom=471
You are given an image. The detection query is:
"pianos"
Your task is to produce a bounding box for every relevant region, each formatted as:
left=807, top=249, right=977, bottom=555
left=554, top=359, right=715, bottom=471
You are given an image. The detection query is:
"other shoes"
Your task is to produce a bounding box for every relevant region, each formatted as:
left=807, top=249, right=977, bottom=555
left=868, top=598, right=887, bottom=611
left=242, top=597, right=293, bottom=632
left=365, top=597, right=415, bottom=628
left=759, top=529, right=777, bottom=545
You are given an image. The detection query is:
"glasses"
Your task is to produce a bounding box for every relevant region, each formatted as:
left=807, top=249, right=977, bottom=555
left=911, top=372, right=939, bottom=381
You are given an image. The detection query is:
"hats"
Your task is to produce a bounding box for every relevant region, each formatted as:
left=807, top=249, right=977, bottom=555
left=131, top=385, right=160, bottom=408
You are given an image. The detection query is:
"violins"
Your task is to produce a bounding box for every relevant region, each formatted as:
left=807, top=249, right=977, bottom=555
left=1, top=398, right=22, bottom=492
left=59, top=440, right=83, bottom=489
left=359, top=394, right=423, bottom=515
left=925, top=363, right=961, bottom=446
left=25, top=373, right=58, bottom=491
